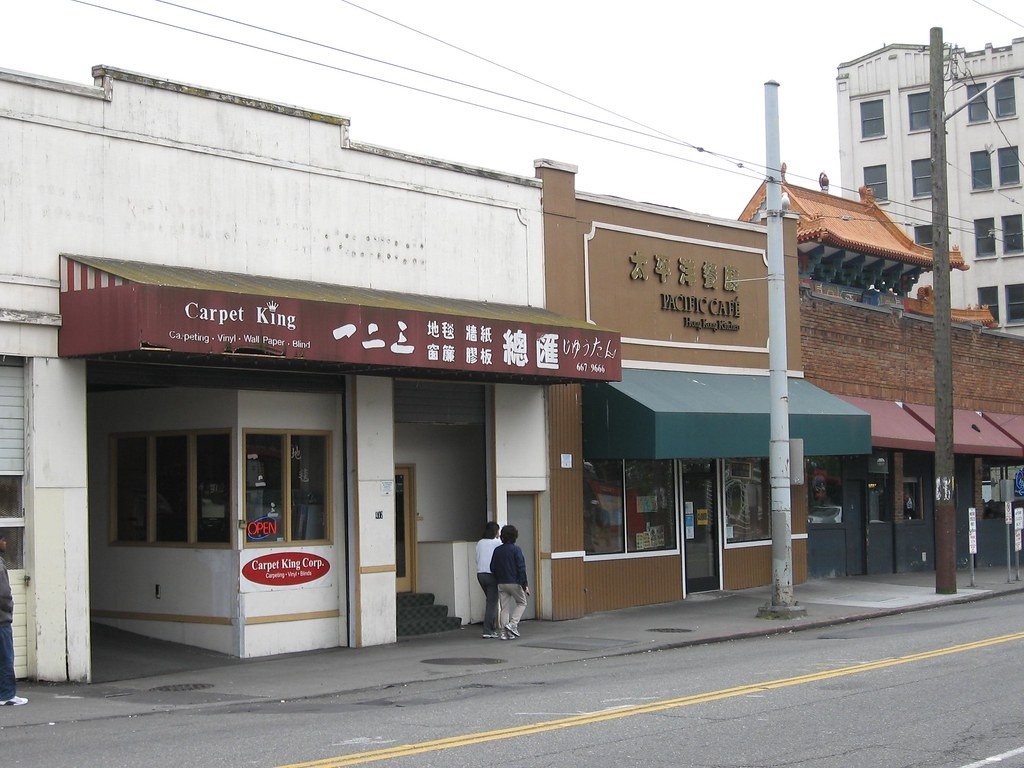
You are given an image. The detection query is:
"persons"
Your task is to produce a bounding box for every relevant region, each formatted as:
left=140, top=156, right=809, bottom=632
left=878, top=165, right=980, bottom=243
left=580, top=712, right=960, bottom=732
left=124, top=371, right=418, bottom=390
left=0, top=533, right=28, bottom=707
left=474, top=521, right=504, bottom=639
left=489, top=525, right=531, bottom=641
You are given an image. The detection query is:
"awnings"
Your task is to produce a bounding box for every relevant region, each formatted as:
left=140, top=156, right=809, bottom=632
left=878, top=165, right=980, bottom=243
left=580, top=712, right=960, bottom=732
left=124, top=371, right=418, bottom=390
left=55, top=252, right=624, bottom=386
left=582, top=368, right=873, bottom=460
left=831, top=393, right=1024, bottom=459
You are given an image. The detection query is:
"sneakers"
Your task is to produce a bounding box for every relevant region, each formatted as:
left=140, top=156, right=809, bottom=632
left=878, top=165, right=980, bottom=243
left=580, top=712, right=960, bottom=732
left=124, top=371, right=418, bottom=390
left=1, top=695, right=29, bottom=706
left=483, top=631, right=499, bottom=638
left=501, top=633, right=515, bottom=640
left=505, top=623, right=521, bottom=637
